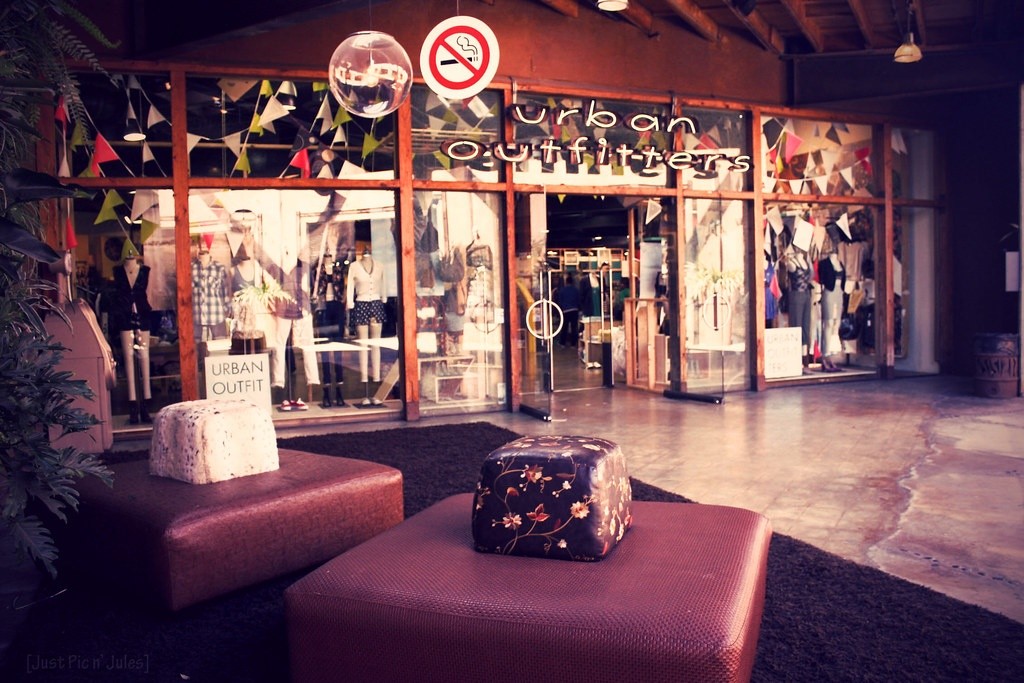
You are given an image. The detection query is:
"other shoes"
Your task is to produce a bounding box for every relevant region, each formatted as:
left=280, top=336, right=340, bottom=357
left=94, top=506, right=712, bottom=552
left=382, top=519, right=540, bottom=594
left=557, top=341, right=565, bottom=349
left=362, top=398, right=371, bottom=406
left=570, top=344, right=576, bottom=349
left=373, top=397, right=382, bottom=406
left=822, top=363, right=842, bottom=372
left=803, top=367, right=813, bottom=375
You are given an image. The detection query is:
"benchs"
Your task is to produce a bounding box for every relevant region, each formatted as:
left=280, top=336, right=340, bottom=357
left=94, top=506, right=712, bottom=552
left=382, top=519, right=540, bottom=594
left=30, top=450, right=404, bottom=618
left=282, top=489, right=773, bottom=683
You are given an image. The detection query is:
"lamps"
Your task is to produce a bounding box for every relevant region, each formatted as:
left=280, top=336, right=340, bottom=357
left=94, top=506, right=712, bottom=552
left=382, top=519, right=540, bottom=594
left=892, top=7, right=922, bottom=63
left=328, top=4, right=413, bottom=119
left=596, top=0, right=627, bottom=12
left=122, top=115, right=146, bottom=141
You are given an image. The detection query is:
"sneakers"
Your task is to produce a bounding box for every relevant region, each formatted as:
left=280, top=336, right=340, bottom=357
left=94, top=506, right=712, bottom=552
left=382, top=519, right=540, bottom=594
left=280, top=400, right=291, bottom=410
left=290, top=397, right=309, bottom=410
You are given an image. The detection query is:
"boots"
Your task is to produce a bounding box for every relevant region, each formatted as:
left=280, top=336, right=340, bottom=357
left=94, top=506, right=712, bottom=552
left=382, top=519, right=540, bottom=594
left=128, top=401, right=139, bottom=424
left=139, top=400, right=153, bottom=423
left=323, top=386, right=332, bottom=408
left=335, top=386, right=345, bottom=407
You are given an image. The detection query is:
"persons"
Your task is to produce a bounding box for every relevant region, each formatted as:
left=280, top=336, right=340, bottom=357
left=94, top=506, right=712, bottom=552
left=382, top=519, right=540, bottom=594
left=268, top=256, right=322, bottom=411
left=618, top=277, right=629, bottom=310
left=762, top=247, right=781, bottom=329
left=345, top=254, right=388, bottom=406
left=555, top=276, right=581, bottom=349
left=189, top=250, right=232, bottom=373
left=229, top=258, right=267, bottom=353
left=113, top=256, right=154, bottom=424
left=595, top=261, right=610, bottom=301
left=266, top=256, right=321, bottom=412
left=787, top=256, right=818, bottom=375
left=654, top=271, right=668, bottom=324
left=659, top=289, right=673, bottom=381
left=818, top=257, right=848, bottom=372
left=580, top=276, right=606, bottom=369
left=310, top=261, right=347, bottom=392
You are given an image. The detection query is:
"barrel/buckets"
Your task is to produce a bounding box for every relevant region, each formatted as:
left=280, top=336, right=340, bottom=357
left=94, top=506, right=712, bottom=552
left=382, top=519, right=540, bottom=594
left=974, top=331, right=1019, bottom=398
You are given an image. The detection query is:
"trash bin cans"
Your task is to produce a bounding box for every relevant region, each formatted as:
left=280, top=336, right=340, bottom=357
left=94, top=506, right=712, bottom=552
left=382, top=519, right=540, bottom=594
left=974, top=332, right=1019, bottom=398
